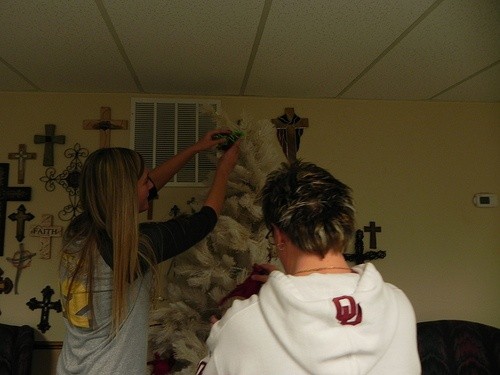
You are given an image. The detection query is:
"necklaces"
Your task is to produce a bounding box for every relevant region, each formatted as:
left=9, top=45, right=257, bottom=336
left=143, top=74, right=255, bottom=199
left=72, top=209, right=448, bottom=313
left=290, top=266, right=354, bottom=276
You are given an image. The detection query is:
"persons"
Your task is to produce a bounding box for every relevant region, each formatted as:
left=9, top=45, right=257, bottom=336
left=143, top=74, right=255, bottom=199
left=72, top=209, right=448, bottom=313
left=196, top=159, right=422, bottom=375
left=55, top=130, right=245, bottom=375
